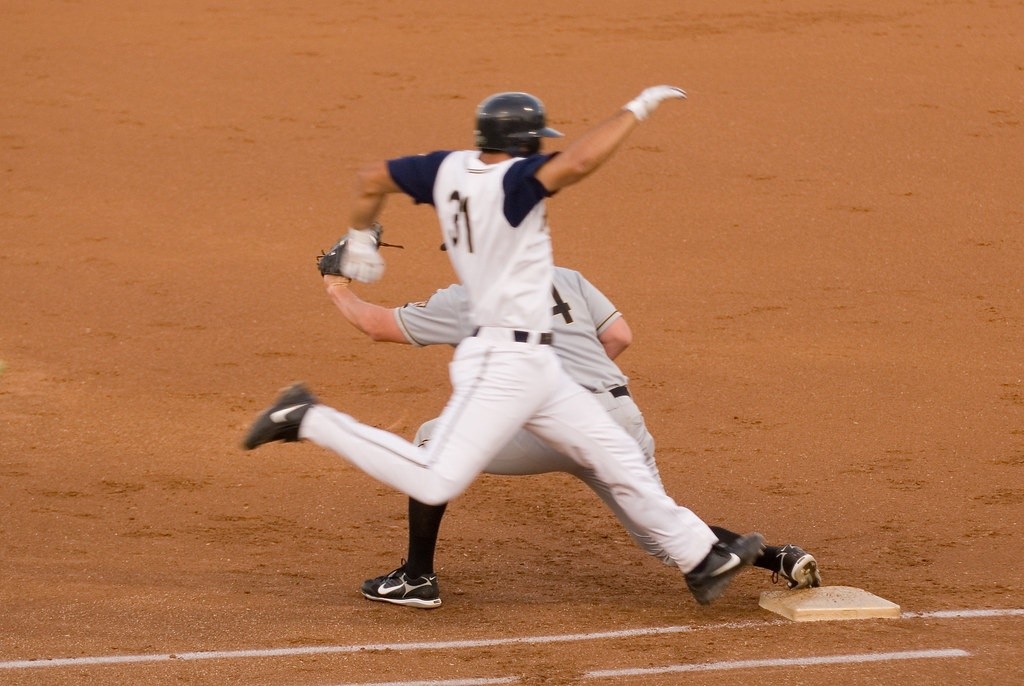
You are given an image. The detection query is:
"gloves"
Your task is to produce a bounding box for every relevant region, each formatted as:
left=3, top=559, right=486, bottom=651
left=340, top=229, right=385, bottom=281
left=622, top=83, right=688, bottom=121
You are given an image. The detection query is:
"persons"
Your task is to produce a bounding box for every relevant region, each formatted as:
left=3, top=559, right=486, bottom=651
left=318, top=222, right=823, bottom=610
left=241, top=86, right=764, bottom=605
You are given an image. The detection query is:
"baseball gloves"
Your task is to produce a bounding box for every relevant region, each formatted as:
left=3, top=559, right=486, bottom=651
left=316, top=222, right=385, bottom=283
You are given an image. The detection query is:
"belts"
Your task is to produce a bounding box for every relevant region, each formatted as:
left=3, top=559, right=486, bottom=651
left=610, top=385, right=631, bottom=398
left=513, top=326, right=555, bottom=344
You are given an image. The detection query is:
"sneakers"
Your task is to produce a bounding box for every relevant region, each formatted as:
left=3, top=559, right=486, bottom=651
left=778, top=544, right=821, bottom=591
left=360, top=564, right=444, bottom=608
left=240, top=383, right=312, bottom=448
left=684, top=530, right=766, bottom=605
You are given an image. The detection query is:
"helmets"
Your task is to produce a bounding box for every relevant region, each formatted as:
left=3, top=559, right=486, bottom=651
left=474, top=90, right=565, bottom=152
